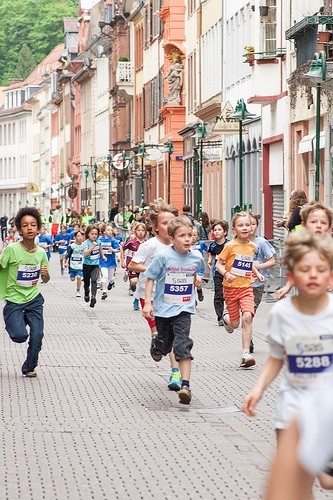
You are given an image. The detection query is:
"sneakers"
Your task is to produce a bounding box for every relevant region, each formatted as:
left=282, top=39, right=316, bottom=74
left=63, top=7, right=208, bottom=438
left=223, top=309, right=234, bottom=333
left=238, top=351, right=255, bottom=368
left=151, top=335, right=162, bottom=361
left=168, top=372, right=182, bottom=391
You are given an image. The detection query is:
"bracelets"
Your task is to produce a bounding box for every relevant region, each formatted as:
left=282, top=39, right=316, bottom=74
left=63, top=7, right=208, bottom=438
left=224, top=271, right=228, bottom=277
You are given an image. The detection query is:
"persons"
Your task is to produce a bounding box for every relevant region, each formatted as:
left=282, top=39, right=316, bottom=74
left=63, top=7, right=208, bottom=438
left=209, top=219, right=231, bottom=325
left=275, top=201, right=333, bottom=300
left=276, top=189, right=310, bottom=234
left=143, top=215, right=206, bottom=404
left=160, top=52, right=184, bottom=106
left=128, top=198, right=181, bottom=391
left=0, top=206, right=50, bottom=377
left=250, top=212, right=277, bottom=351
left=266, top=364, right=333, bottom=500
left=242, top=230, right=333, bottom=493
left=215, top=211, right=266, bottom=367
left=1, top=204, right=208, bottom=311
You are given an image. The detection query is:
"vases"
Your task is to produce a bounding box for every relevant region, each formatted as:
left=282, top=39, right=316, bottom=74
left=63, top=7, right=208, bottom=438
left=319, top=32, right=331, bottom=41
left=317, top=44, right=325, bottom=52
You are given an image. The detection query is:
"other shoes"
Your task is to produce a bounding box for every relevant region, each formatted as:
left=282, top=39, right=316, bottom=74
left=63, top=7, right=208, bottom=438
left=133, top=299, right=139, bottom=310
left=84, top=296, right=89, bottom=302
left=219, top=320, right=224, bottom=326
left=129, top=289, right=133, bottom=296
left=76, top=291, right=81, bottom=297
left=90, top=298, right=96, bottom=307
left=197, top=288, right=204, bottom=301
left=26, top=369, right=37, bottom=377
left=102, top=292, right=107, bottom=300
left=250, top=339, right=254, bottom=353
left=178, top=386, right=192, bottom=404
left=97, top=281, right=101, bottom=288
left=108, top=282, right=114, bottom=290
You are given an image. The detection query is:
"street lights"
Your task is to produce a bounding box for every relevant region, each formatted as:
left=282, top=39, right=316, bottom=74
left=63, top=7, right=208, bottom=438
left=225, top=98, right=256, bottom=211
left=118, top=149, right=133, bottom=227
left=102, top=153, right=116, bottom=222
left=160, top=138, right=176, bottom=204
left=133, top=143, right=152, bottom=208
left=191, top=120, right=208, bottom=222
left=83, top=168, right=90, bottom=215
left=69, top=174, right=76, bottom=224
left=302, top=50, right=333, bottom=207
left=91, top=162, right=99, bottom=218
